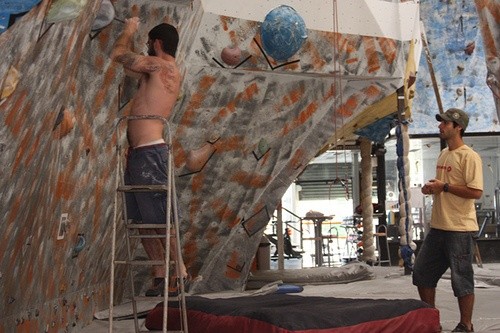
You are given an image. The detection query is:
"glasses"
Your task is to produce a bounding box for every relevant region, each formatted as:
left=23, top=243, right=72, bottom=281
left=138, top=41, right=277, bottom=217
left=146, top=40, right=151, bottom=48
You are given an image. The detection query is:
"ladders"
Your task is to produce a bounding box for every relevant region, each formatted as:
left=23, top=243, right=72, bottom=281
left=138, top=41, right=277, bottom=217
left=108, top=114, right=189, bottom=333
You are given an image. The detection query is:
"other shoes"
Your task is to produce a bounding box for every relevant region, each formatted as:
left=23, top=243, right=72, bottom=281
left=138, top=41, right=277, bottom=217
left=161, top=273, right=188, bottom=296
left=146, top=278, right=170, bottom=295
left=451, top=322, right=473, bottom=333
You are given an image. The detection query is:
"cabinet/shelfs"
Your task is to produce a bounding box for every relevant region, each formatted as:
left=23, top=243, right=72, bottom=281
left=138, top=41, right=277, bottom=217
left=372, top=224, right=391, bottom=267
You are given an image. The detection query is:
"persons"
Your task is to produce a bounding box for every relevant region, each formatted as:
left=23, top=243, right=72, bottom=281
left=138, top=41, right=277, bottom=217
left=109, top=16, right=191, bottom=297
left=411, top=108, right=483, bottom=333
left=282, top=229, right=303, bottom=259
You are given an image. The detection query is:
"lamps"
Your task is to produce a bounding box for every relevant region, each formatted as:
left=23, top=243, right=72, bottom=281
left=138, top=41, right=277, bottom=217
left=331, top=0, right=351, bottom=185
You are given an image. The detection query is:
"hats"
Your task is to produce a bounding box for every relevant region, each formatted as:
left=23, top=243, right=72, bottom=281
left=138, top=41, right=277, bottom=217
left=436, top=107, right=469, bottom=131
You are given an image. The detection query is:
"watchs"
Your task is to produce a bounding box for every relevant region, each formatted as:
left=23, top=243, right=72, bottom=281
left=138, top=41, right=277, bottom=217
left=443, top=182, right=450, bottom=193
